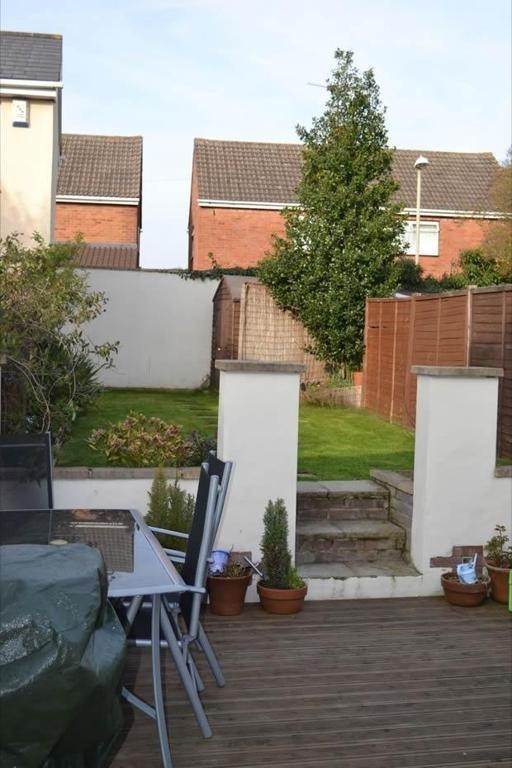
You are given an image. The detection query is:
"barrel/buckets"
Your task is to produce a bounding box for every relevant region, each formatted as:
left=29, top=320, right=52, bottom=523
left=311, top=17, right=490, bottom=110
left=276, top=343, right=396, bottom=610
left=209, top=550, right=231, bottom=574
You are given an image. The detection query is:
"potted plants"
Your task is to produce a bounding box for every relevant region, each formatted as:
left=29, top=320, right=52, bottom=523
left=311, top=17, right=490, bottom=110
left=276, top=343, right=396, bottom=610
left=484, top=523, right=512, bottom=604
left=255, top=496, right=306, bottom=614
left=207, top=545, right=253, bottom=615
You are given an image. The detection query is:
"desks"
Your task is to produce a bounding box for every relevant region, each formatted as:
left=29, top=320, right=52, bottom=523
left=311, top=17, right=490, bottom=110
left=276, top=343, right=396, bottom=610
left=1, top=507, right=185, bottom=767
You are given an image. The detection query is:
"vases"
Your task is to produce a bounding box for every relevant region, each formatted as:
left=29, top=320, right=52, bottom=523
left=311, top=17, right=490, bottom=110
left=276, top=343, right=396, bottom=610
left=351, top=371, right=363, bottom=385
left=440, top=572, right=492, bottom=607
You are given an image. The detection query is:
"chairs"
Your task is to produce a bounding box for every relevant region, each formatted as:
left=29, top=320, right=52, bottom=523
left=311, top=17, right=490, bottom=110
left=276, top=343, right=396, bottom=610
left=0, top=431, right=53, bottom=509
left=120, top=448, right=234, bottom=692
left=116, top=461, right=220, bottom=768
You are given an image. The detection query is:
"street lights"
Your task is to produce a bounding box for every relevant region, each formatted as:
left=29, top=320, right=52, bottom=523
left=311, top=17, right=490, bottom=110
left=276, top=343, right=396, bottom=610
left=414, top=153, right=430, bottom=263
